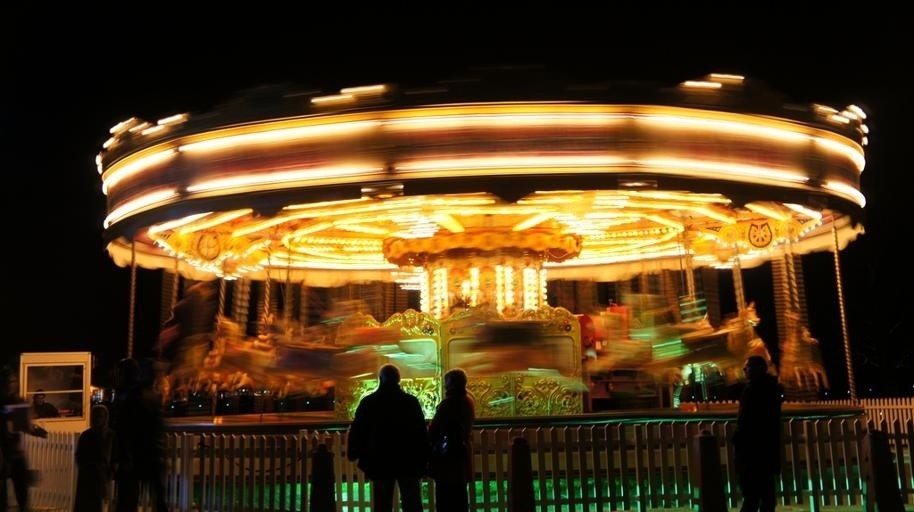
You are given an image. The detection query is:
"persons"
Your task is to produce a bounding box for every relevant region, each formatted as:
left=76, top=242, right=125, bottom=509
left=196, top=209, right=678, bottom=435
left=347, top=366, right=427, bottom=512
left=28, top=390, right=59, bottom=419
left=0, top=364, right=47, bottom=512
left=72, top=405, right=119, bottom=512
left=719, top=356, right=783, bottom=512
left=111, top=356, right=168, bottom=512
left=426, top=371, right=475, bottom=512
